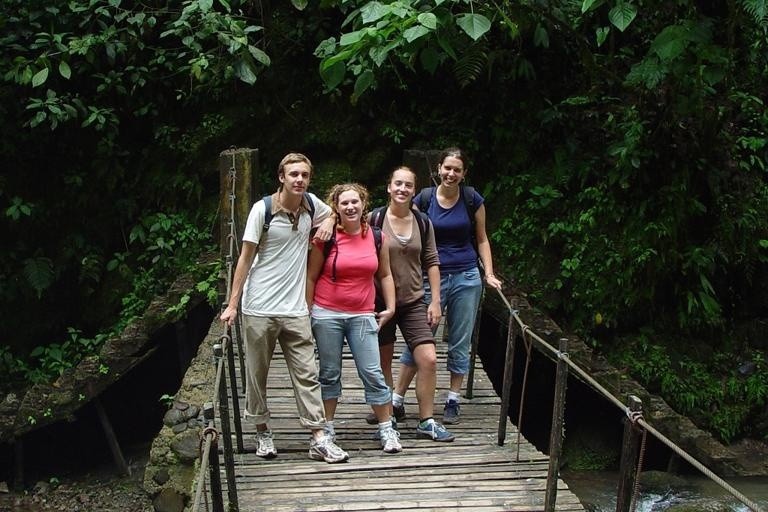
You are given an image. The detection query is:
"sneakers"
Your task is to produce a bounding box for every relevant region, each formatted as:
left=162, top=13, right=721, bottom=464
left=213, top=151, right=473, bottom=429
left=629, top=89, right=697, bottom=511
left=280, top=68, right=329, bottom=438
left=366, top=404, right=405, bottom=422
left=443, top=400, right=461, bottom=425
left=374, top=416, right=398, bottom=436
left=308, top=436, right=348, bottom=465
left=325, top=430, right=338, bottom=444
left=254, top=432, right=280, bottom=460
left=376, top=429, right=403, bottom=454
left=416, top=423, right=455, bottom=442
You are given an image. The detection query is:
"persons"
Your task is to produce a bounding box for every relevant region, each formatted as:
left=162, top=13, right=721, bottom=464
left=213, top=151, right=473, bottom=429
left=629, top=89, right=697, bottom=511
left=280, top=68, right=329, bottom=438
left=218, top=152, right=351, bottom=464
left=306, top=181, right=405, bottom=454
left=365, top=144, right=504, bottom=425
left=360, top=163, right=457, bottom=444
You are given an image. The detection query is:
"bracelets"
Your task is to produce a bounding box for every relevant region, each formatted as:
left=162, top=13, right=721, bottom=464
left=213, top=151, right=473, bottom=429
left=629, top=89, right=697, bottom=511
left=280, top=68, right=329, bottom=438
left=485, top=274, right=495, bottom=278
